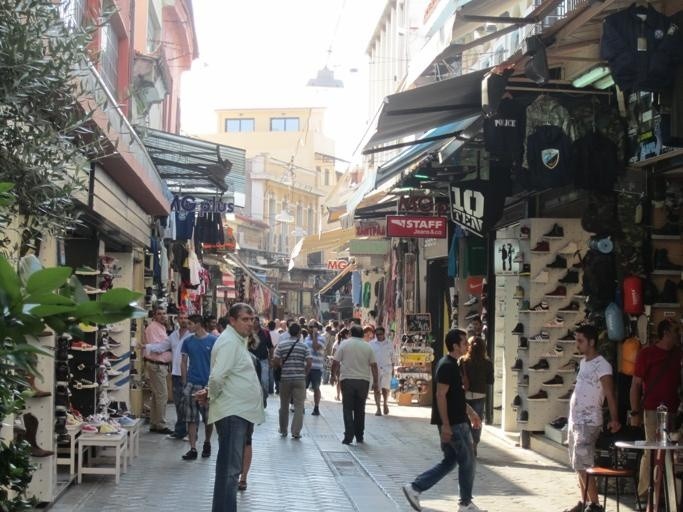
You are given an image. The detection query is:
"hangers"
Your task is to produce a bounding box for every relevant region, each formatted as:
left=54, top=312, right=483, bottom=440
left=602, top=2, right=682, bottom=25
left=490, top=88, right=611, bottom=139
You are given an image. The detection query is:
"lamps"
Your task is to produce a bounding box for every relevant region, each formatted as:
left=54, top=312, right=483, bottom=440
left=480, top=66, right=560, bottom=117
left=409, top=164, right=434, bottom=184
left=517, top=34, right=556, bottom=84
left=147, top=145, right=235, bottom=195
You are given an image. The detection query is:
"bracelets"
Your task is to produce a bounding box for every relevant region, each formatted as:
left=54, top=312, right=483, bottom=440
left=630, top=409, right=638, bottom=417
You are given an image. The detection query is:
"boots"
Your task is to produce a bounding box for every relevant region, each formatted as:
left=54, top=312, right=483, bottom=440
left=13, top=353, right=55, bottom=458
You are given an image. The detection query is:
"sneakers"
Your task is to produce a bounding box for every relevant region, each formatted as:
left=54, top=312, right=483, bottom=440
left=238, top=480, right=247, bottom=489
left=64, top=398, right=137, bottom=435
left=462, top=295, right=480, bottom=320
left=562, top=500, right=605, bottom=512
left=650, top=222, right=683, bottom=304
left=512, top=222, right=584, bottom=427
left=341, top=436, right=364, bottom=443
left=374, top=405, right=389, bottom=415
left=202, top=442, right=212, bottom=457
left=456, top=501, right=488, bottom=512
left=402, top=483, right=423, bottom=512
left=148, top=424, right=189, bottom=441
left=311, top=410, right=320, bottom=415
left=279, top=431, right=302, bottom=438
left=96, top=322, right=123, bottom=392
left=182, top=449, right=198, bottom=460
left=69, top=265, right=102, bottom=390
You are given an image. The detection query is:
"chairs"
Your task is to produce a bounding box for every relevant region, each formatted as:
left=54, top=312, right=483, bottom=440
left=581, top=420, right=642, bottom=511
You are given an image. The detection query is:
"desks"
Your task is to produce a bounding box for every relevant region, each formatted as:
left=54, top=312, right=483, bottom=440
left=612, top=438, right=682, bottom=512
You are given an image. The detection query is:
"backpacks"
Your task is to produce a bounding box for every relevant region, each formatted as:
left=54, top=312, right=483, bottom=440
left=579, top=188, right=652, bottom=384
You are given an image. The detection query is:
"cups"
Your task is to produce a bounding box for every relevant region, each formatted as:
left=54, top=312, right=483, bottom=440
left=670, top=431, right=680, bottom=446
left=655, top=411, right=668, bottom=446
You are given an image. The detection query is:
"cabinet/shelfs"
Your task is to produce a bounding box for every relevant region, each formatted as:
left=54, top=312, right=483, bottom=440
left=0, top=200, right=53, bottom=509
left=489, top=216, right=585, bottom=449
left=648, top=188, right=682, bottom=344
left=53, top=249, right=140, bottom=487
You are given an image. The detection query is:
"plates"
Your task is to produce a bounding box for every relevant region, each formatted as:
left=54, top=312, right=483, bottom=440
left=635, top=441, right=647, bottom=445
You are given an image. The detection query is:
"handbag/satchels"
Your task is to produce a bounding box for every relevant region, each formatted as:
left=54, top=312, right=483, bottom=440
left=273, top=366, right=281, bottom=382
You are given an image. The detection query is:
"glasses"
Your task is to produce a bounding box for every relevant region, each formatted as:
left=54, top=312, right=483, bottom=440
left=375, top=333, right=384, bottom=336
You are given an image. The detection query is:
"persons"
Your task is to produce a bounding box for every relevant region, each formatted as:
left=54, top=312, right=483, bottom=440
left=457, top=335, right=494, bottom=459
left=561, top=323, right=624, bottom=512
left=142, top=301, right=396, bottom=462
left=188, top=302, right=267, bottom=511
left=235, top=427, right=254, bottom=489
left=471, top=316, right=482, bottom=336
left=399, top=326, right=489, bottom=511
left=628, top=315, right=683, bottom=512
